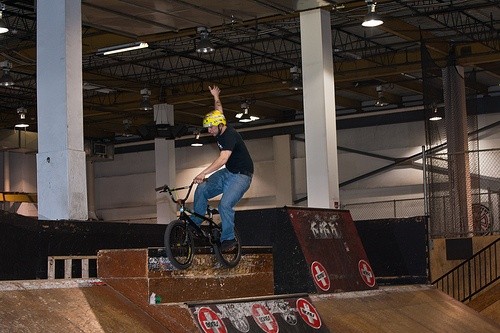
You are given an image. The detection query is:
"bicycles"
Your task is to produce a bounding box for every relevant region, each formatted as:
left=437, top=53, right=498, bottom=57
left=155, top=178, right=242, bottom=269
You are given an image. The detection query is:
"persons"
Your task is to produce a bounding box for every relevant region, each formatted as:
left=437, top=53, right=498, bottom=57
left=186, top=85, right=253, bottom=251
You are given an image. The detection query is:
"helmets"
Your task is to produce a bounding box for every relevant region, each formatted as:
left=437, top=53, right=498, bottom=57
left=203, top=110, right=227, bottom=126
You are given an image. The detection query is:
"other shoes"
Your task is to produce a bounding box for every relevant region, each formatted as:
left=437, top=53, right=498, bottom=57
left=221, top=238, right=237, bottom=250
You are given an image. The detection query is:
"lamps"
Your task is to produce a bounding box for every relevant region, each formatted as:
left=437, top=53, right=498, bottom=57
left=14, top=106, right=30, bottom=129
left=361, top=0, right=384, bottom=28
left=374, top=86, right=389, bottom=107
left=429, top=102, right=443, bottom=121
left=0, top=60, right=15, bottom=87
left=138, top=88, right=153, bottom=111
left=287, top=67, right=302, bottom=90
left=195, top=26, right=216, bottom=53
left=237, top=95, right=256, bottom=123
